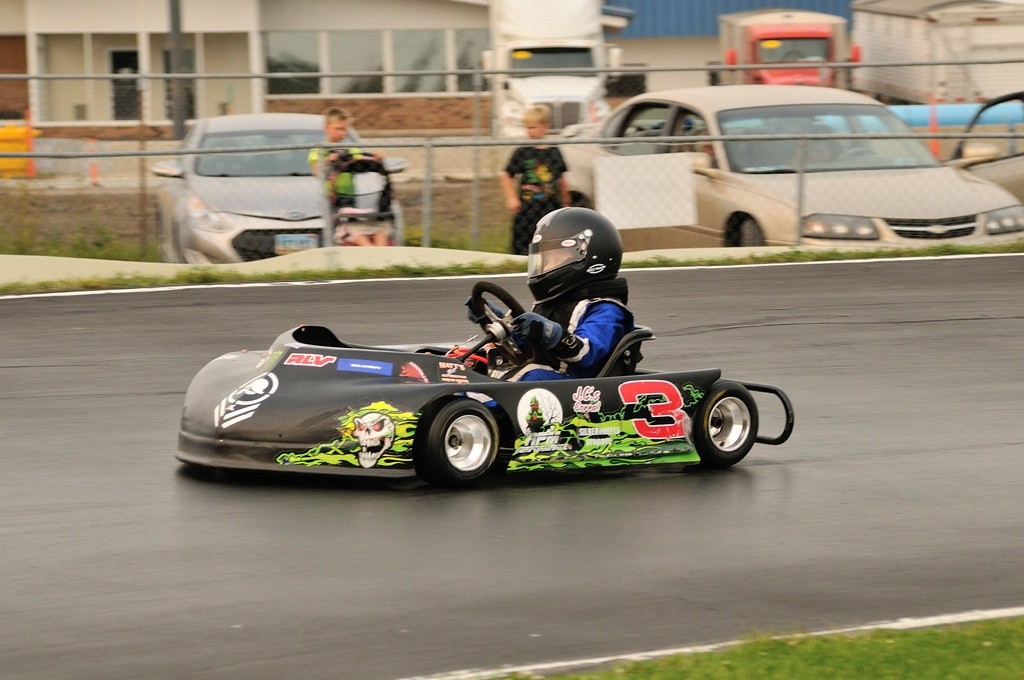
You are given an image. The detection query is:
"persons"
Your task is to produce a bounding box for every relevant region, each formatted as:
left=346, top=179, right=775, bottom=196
left=309, top=107, right=389, bottom=246
left=804, top=125, right=832, bottom=163
left=464, top=206, right=634, bottom=381
left=499, top=102, right=572, bottom=256
left=695, top=130, right=725, bottom=168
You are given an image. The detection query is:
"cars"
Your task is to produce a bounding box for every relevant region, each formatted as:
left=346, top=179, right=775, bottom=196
left=547, top=85, right=1024, bottom=255
left=150, top=113, right=407, bottom=267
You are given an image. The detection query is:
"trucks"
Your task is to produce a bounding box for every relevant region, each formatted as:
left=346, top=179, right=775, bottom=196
left=718, top=9, right=863, bottom=93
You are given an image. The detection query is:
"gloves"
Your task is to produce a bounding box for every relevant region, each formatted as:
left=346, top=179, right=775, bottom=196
left=465, top=293, right=505, bottom=332
left=508, top=312, right=562, bottom=354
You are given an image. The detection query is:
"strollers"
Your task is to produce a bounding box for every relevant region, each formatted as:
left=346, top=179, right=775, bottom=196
left=328, top=153, right=397, bottom=246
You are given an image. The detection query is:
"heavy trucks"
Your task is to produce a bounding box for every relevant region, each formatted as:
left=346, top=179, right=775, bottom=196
left=482, top=1, right=623, bottom=152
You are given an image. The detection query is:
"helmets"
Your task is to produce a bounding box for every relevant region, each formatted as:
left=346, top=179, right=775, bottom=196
left=527, top=206, right=623, bottom=314
